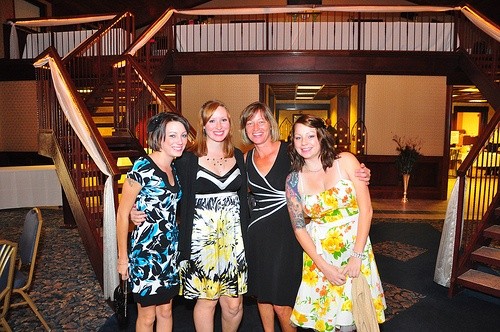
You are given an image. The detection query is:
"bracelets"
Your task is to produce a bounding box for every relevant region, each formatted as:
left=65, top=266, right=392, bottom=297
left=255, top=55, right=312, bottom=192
left=349, top=249, right=367, bottom=260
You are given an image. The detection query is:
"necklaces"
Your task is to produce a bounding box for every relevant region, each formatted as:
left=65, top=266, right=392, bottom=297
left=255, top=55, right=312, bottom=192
left=202, top=151, right=227, bottom=168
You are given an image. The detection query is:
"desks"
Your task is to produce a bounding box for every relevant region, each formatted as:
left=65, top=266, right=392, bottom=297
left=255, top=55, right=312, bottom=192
left=172, top=22, right=460, bottom=51
left=0, top=160, right=100, bottom=210
left=462, top=152, right=500, bottom=178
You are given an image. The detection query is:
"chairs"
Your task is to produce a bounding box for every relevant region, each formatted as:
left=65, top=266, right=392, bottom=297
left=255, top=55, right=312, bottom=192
left=0, top=208, right=51, bottom=332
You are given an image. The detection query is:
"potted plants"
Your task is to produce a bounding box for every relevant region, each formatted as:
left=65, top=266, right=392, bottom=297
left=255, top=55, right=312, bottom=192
left=392, top=134, right=425, bottom=202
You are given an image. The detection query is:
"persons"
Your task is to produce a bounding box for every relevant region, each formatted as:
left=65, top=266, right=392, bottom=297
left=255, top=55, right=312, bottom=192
left=285, top=114, right=387, bottom=332
left=116, top=111, right=190, bottom=332
left=239, top=101, right=371, bottom=332
left=134, top=103, right=160, bottom=148
left=129, top=100, right=249, bottom=332
